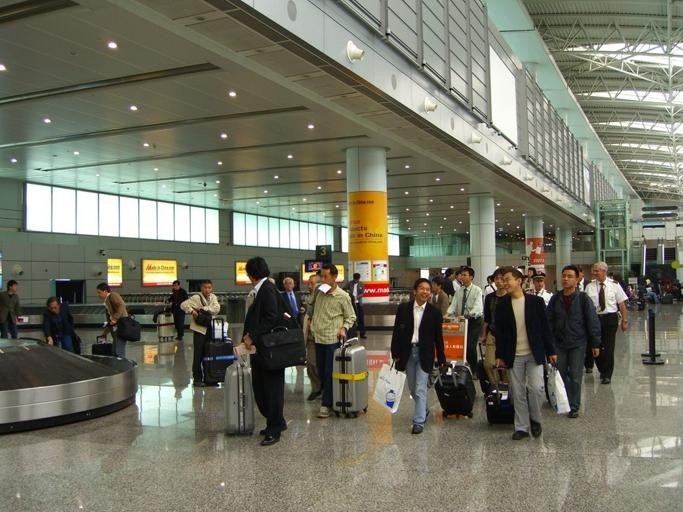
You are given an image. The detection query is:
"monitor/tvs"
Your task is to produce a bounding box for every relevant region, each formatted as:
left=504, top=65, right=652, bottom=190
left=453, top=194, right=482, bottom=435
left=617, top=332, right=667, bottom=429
left=305, top=260, right=324, bottom=272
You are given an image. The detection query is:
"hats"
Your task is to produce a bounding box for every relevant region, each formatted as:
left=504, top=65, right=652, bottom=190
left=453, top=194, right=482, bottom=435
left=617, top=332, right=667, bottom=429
left=531, top=271, right=546, bottom=281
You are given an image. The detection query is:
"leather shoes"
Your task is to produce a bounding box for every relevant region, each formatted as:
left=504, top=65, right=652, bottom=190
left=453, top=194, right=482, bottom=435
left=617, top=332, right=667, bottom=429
left=512, top=431, right=529, bottom=440
left=530, top=415, right=542, bottom=437
left=261, top=432, right=280, bottom=446
left=260, top=418, right=288, bottom=435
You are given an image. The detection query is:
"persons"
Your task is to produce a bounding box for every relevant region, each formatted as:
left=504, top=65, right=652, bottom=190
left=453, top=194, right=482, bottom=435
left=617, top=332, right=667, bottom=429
left=390, top=261, right=630, bottom=439
left=96, top=283, right=129, bottom=358
left=165, top=281, right=189, bottom=341
left=628, top=273, right=682, bottom=312
left=42, top=296, right=75, bottom=352
left=0, top=280, right=23, bottom=339
left=241, top=257, right=367, bottom=445
left=179, top=280, right=221, bottom=387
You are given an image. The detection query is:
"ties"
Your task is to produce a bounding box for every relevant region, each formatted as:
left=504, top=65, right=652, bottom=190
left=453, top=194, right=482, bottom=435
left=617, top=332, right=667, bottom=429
left=461, top=288, right=467, bottom=315
left=535, top=291, right=539, bottom=295
left=599, top=283, right=605, bottom=311
left=289, top=292, right=297, bottom=314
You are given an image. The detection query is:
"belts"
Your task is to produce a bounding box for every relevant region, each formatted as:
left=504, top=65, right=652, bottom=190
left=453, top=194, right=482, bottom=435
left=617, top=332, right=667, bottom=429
left=411, top=343, right=419, bottom=347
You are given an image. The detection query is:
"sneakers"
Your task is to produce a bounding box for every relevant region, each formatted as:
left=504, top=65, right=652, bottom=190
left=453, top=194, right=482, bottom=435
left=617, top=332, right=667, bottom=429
left=586, top=368, right=593, bottom=373
left=568, top=408, right=579, bottom=418
left=412, top=409, right=430, bottom=434
left=317, top=406, right=334, bottom=418
left=603, top=377, right=611, bottom=384
left=307, top=391, right=322, bottom=402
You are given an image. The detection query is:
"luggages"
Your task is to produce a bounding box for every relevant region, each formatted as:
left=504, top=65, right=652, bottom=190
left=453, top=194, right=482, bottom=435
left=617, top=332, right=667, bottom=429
left=434, top=361, right=476, bottom=418
left=224, top=339, right=255, bottom=435
left=157, top=300, right=176, bottom=339
left=478, top=342, right=514, bottom=424
left=202, top=318, right=235, bottom=383
left=332, top=335, right=369, bottom=418
left=92, top=335, right=112, bottom=356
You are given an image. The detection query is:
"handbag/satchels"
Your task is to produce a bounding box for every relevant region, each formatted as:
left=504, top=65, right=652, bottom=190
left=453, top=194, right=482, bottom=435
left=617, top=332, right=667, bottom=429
left=118, top=317, right=140, bottom=342
left=372, top=363, right=406, bottom=414
left=195, top=309, right=211, bottom=327
left=547, top=363, right=571, bottom=414
left=257, top=328, right=306, bottom=372
